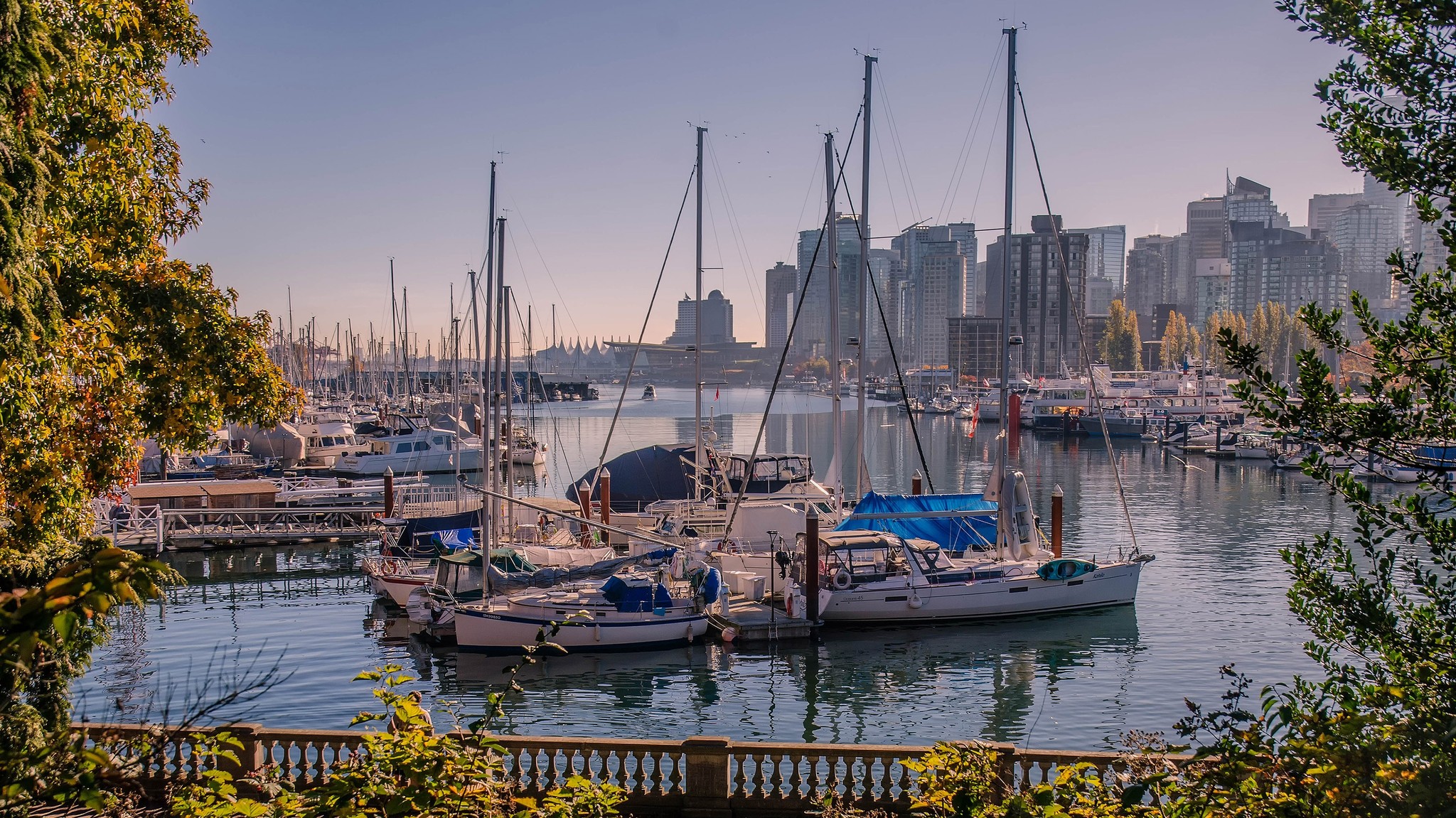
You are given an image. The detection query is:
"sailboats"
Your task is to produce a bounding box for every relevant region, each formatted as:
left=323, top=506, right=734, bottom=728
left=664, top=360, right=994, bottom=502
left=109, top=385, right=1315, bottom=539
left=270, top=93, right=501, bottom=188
left=786, top=610, right=1138, bottom=741
left=105, top=24, right=1453, bottom=651
left=435, top=640, right=744, bottom=710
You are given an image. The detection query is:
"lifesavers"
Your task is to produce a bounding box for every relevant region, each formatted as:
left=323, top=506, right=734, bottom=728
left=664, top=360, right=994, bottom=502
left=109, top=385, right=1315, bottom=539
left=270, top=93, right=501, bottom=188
left=792, top=484, right=805, bottom=494
left=833, top=571, right=851, bottom=590
left=717, top=538, right=736, bottom=553
left=1119, top=412, right=1125, bottom=418
left=1217, top=407, right=1223, bottom=413
left=382, top=562, right=397, bottom=574
